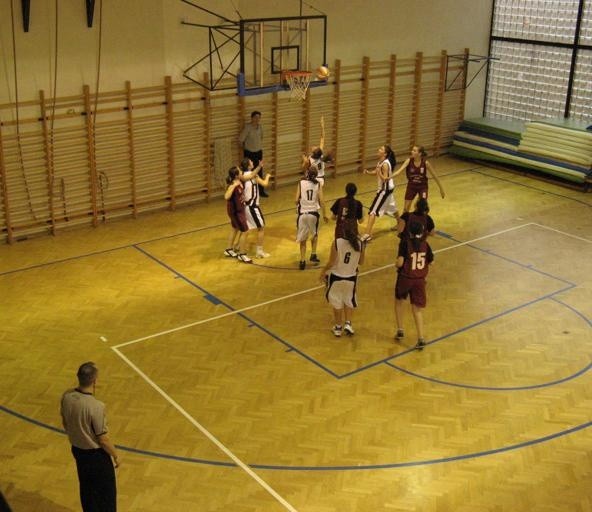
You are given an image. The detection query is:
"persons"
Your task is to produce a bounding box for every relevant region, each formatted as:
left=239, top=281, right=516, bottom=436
left=385, top=144, right=445, bottom=213
left=395, top=222, right=434, bottom=350
left=301, top=115, right=335, bottom=188
left=61, top=361, right=120, bottom=512
left=397, top=199, right=435, bottom=239
left=331, top=183, right=363, bottom=238
left=224, top=158, right=266, bottom=263
left=239, top=110, right=269, bottom=197
left=295, top=167, right=329, bottom=270
left=320, top=217, right=364, bottom=336
left=234, top=157, right=271, bottom=259
left=360, top=145, right=401, bottom=242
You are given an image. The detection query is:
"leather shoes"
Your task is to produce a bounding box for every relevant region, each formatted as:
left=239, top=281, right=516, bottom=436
left=260, top=189, right=269, bottom=197
left=300, top=254, right=320, bottom=270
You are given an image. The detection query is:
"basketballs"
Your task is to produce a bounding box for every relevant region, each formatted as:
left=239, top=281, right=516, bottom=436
left=316, top=66, right=330, bottom=80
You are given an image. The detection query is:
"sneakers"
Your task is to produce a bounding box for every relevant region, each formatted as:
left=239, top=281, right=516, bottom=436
left=395, top=328, right=426, bottom=349
left=331, top=321, right=354, bottom=337
left=224, top=244, right=271, bottom=263
left=360, top=234, right=372, bottom=241
